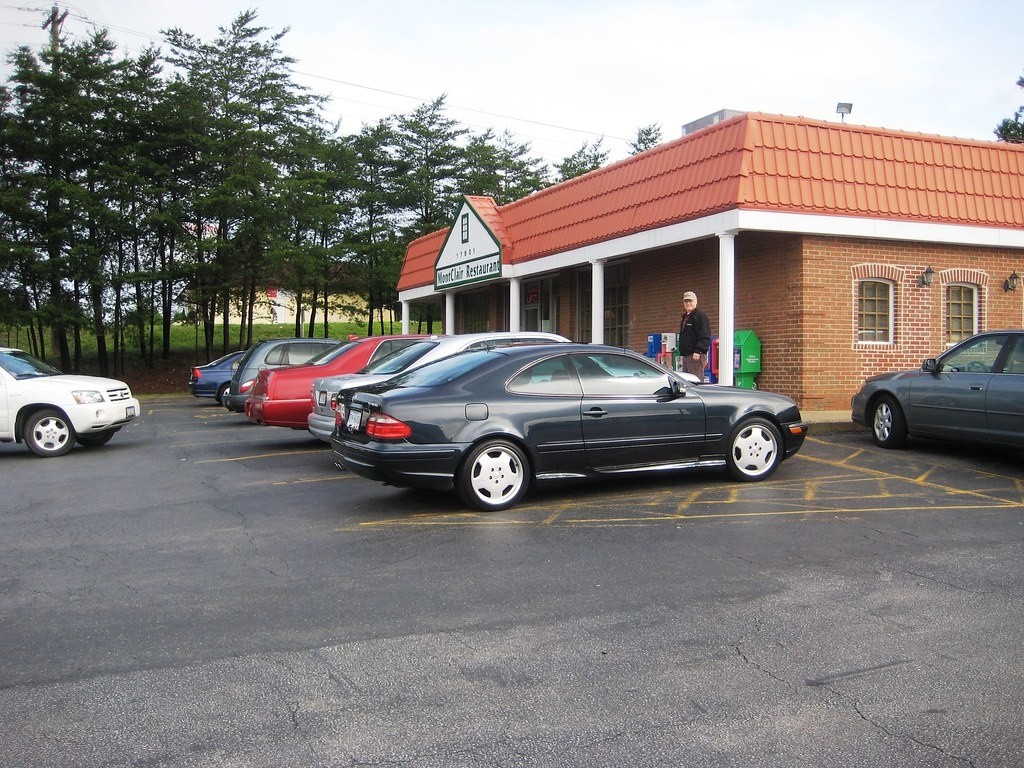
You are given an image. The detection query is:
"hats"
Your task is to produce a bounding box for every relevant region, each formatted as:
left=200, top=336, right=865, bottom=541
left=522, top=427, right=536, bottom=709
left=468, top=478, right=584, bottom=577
left=683, top=291, right=696, bottom=301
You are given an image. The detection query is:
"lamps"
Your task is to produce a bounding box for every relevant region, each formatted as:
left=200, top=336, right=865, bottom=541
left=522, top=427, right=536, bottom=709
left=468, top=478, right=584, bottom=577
left=836, top=102, right=853, bottom=123
left=1003, top=270, right=1020, bottom=292
left=918, top=265, right=935, bottom=289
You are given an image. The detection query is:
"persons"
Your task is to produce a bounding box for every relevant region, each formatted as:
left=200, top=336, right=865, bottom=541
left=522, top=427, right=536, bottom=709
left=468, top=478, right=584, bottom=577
left=677, top=291, right=711, bottom=383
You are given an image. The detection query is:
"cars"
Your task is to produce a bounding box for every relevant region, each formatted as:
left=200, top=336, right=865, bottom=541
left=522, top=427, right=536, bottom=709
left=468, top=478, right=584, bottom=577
left=189, top=351, right=248, bottom=405
left=308, top=330, right=702, bottom=446
left=222, top=338, right=345, bottom=412
left=244, top=333, right=448, bottom=433
left=328, top=340, right=809, bottom=518
left=848, top=330, right=1024, bottom=452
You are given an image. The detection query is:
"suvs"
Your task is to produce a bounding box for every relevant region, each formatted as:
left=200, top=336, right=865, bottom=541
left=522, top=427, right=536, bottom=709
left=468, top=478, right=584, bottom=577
left=1, top=347, right=140, bottom=460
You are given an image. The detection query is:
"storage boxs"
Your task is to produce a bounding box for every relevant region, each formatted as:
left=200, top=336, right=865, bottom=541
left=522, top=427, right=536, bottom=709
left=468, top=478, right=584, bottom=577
left=641, top=332, right=717, bottom=384
